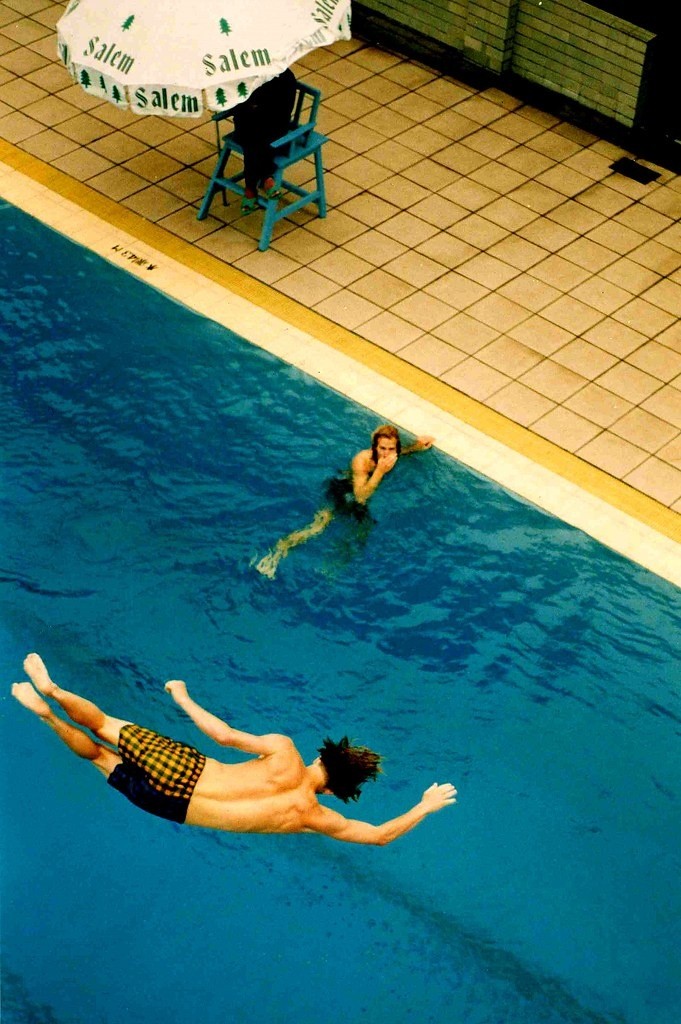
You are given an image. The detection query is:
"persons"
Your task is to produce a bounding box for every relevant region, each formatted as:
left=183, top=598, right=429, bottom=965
left=10, top=653, right=457, bottom=846
left=353, top=424, right=436, bottom=474
left=232, top=69, right=297, bottom=215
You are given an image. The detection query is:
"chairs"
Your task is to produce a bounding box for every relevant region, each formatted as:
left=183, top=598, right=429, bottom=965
left=196, top=78, right=331, bottom=254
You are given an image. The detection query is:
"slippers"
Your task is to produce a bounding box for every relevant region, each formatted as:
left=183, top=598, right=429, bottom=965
left=241, top=196, right=258, bottom=215
left=266, top=185, right=283, bottom=200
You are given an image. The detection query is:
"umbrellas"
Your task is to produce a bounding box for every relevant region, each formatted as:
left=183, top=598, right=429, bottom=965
left=56, top=0, right=352, bottom=118
